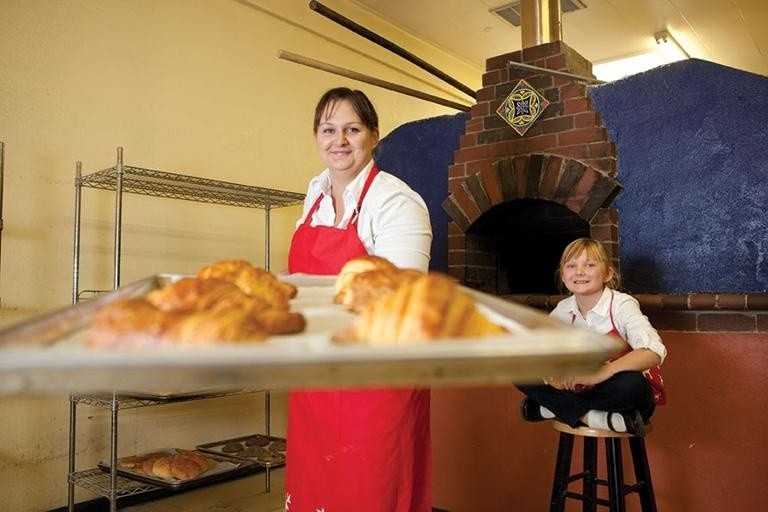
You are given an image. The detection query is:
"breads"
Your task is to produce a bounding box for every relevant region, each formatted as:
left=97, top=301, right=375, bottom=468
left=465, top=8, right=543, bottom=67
left=86, top=253, right=511, bottom=357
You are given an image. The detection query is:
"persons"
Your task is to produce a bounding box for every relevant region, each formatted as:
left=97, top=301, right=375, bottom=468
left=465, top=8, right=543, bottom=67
left=285, top=87, right=433, bottom=512
left=513, top=238, right=667, bottom=437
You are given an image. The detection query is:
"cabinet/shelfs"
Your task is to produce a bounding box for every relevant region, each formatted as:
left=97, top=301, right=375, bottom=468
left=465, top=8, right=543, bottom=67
left=67, top=147, right=307, bottom=512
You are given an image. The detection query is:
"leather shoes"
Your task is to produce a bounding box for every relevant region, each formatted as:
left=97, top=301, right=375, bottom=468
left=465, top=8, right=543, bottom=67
left=521, top=397, right=557, bottom=421
left=607, top=406, right=647, bottom=438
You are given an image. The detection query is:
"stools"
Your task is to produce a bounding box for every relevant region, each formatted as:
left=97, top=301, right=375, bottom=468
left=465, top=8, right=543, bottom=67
left=550, top=420, right=657, bottom=512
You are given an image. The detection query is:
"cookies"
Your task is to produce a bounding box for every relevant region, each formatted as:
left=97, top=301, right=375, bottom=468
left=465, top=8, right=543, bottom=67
left=221, top=433, right=289, bottom=465
left=118, top=449, right=210, bottom=483
